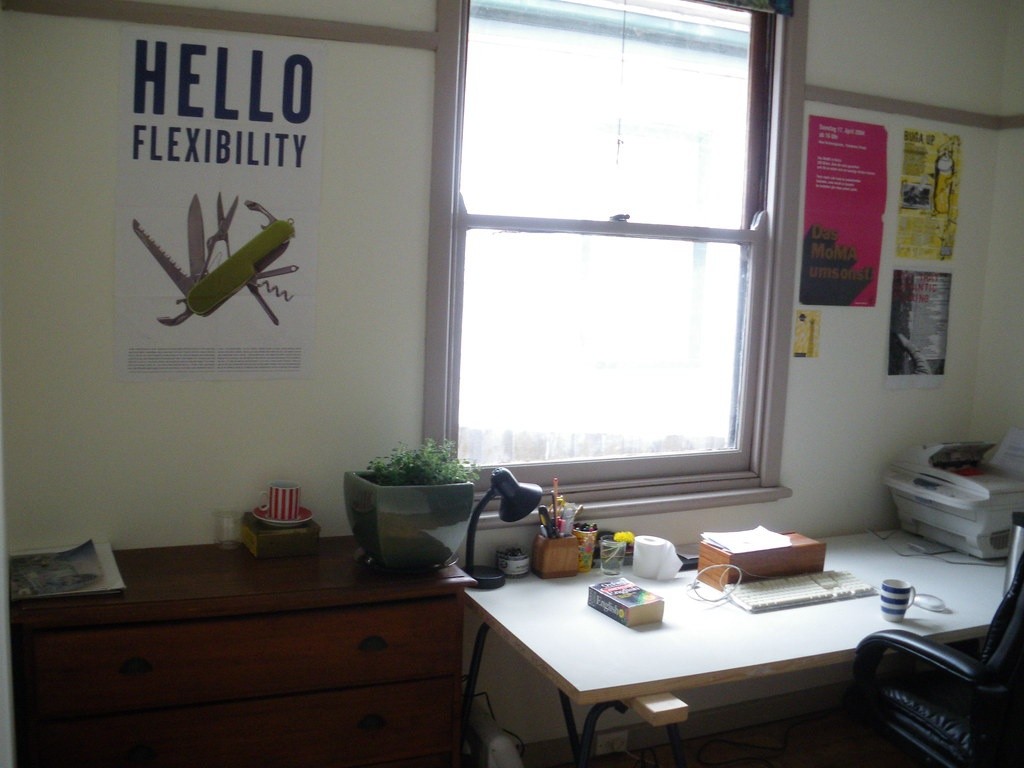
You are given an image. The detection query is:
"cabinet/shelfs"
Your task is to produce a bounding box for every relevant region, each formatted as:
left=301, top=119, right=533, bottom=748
left=5, top=536, right=479, bottom=767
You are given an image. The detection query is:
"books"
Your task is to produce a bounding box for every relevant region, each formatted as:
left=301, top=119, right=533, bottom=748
left=588, top=578, right=665, bottom=628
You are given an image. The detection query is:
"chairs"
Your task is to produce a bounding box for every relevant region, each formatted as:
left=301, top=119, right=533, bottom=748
left=852, top=537, right=1024, bottom=768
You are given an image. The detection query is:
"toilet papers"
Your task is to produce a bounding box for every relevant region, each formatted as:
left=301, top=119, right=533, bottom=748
left=631, top=535, right=683, bottom=580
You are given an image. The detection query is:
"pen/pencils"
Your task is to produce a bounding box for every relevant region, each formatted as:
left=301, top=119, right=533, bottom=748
left=538, top=477, right=583, bottom=538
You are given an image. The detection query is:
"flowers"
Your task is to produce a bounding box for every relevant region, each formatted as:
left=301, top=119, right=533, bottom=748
left=601, top=532, right=634, bottom=565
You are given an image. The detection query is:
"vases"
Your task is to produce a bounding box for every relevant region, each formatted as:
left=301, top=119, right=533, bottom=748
left=601, top=535, right=627, bottom=578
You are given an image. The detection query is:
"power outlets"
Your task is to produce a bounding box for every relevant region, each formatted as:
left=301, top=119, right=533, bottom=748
left=598, top=732, right=628, bottom=754
left=732, top=690, right=756, bottom=710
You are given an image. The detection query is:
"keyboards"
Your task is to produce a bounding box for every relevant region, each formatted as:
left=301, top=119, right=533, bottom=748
left=725, top=571, right=881, bottom=614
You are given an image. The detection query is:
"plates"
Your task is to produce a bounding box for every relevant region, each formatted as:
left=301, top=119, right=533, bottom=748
left=252, top=505, right=314, bottom=527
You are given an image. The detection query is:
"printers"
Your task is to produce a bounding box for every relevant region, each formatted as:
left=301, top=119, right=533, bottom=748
left=882, top=441, right=1024, bottom=559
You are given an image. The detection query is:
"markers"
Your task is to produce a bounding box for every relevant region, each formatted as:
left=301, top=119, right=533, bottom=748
left=538, top=505, right=554, bottom=538
left=573, top=522, right=598, bottom=532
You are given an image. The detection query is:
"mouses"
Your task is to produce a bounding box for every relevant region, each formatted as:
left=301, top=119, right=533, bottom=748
left=914, top=595, right=945, bottom=611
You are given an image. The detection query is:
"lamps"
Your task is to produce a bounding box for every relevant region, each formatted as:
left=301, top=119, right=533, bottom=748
left=460, top=467, right=542, bottom=589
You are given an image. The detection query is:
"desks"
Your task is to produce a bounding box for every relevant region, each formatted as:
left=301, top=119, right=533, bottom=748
left=463, top=530, right=1024, bottom=768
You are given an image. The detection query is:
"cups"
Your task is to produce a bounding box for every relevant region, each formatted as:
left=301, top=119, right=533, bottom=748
left=214, top=511, right=244, bottom=549
left=600, top=535, right=627, bottom=575
left=257, top=480, right=301, bottom=520
left=880, top=579, right=916, bottom=624
left=573, top=529, right=598, bottom=573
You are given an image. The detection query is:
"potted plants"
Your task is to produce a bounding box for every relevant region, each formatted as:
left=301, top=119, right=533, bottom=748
left=344, top=435, right=480, bottom=571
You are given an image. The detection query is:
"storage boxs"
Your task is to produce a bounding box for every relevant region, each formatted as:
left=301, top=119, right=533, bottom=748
left=699, top=534, right=826, bottom=593
